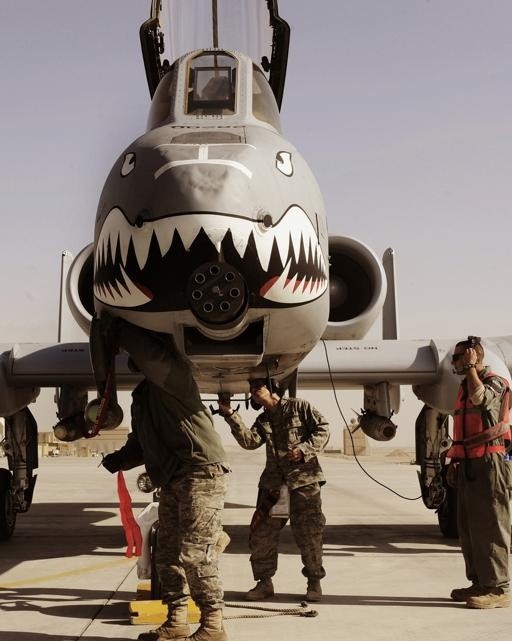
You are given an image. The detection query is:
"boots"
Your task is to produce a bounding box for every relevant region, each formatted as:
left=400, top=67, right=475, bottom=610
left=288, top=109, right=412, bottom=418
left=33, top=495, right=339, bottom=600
left=138, top=605, right=190, bottom=641
left=307, top=578, right=322, bottom=602
left=175, top=609, right=228, bottom=641
left=244, top=578, right=274, bottom=601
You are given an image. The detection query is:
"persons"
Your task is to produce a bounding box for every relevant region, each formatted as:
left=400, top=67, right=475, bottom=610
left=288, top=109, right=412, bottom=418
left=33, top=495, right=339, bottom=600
left=222, top=377, right=330, bottom=602
left=100, top=325, right=235, bottom=640
left=446, top=335, right=511, bottom=609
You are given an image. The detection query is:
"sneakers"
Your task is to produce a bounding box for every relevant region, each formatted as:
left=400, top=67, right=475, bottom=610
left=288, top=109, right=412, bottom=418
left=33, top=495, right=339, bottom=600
left=451, top=583, right=512, bottom=608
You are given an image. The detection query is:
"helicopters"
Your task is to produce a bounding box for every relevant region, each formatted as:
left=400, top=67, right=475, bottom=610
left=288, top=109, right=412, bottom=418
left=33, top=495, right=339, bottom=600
left=1, top=2, right=512, bottom=512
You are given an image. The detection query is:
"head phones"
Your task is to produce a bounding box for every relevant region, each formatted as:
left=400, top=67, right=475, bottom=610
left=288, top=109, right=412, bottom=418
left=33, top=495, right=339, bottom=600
left=452, top=335, right=482, bottom=374
left=250, top=379, right=279, bottom=410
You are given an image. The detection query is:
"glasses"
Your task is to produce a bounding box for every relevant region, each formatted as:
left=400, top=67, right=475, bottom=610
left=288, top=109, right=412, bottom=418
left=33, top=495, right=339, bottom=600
left=453, top=353, right=465, bottom=361
